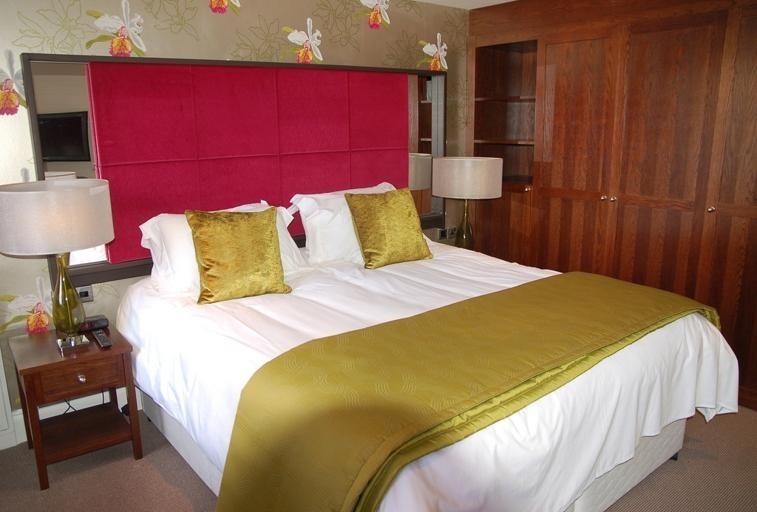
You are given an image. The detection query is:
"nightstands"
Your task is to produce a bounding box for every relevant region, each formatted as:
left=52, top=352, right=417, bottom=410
left=7, top=315, right=144, bottom=490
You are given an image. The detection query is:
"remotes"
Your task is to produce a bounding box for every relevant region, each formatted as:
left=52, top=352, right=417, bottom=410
left=92, top=329, right=112, bottom=348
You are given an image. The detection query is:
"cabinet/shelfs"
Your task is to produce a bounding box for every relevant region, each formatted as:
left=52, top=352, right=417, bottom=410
left=465, top=2, right=754, bottom=412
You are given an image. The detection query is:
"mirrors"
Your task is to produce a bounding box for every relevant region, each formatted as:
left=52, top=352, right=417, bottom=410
left=409, top=70, right=448, bottom=230
left=22, top=53, right=110, bottom=276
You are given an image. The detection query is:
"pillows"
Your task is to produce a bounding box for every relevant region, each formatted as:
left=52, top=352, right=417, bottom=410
left=141, top=179, right=434, bottom=304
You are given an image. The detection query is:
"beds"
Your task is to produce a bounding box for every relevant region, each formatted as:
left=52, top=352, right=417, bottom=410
left=128, top=241, right=687, bottom=511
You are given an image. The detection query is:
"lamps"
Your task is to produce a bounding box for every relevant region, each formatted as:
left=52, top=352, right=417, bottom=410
left=0, top=176, right=115, bottom=337
left=409, top=152, right=430, bottom=191
left=431, top=155, right=502, bottom=250
